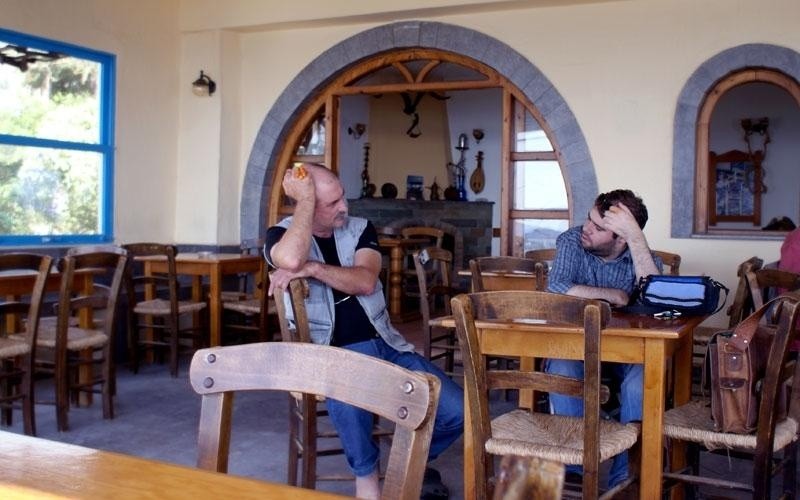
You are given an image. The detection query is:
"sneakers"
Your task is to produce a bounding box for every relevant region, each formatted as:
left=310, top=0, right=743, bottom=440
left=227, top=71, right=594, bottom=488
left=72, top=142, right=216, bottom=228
left=414, top=468, right=450, bottom=499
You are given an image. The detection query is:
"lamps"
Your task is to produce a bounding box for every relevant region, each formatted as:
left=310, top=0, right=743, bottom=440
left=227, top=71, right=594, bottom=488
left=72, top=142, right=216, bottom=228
left=189, top=68, right=217, bottom=99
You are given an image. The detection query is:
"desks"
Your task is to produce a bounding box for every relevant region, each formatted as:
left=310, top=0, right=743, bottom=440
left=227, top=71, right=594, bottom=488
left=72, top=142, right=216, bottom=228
left=0, top=265, right=109, bottom=408
left=0, top=430, right=354, bottom=500
left=132, top=247, right=265, bottom=368
left=428, top=296, right=714, bottom=500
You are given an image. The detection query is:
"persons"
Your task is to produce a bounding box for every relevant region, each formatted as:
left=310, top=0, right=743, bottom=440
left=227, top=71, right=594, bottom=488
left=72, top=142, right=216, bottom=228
left=544, top=189, right=663, bottom=489
left=265, top=160, right=465, bottom=499
left=772, top=226, right=800, bottom=359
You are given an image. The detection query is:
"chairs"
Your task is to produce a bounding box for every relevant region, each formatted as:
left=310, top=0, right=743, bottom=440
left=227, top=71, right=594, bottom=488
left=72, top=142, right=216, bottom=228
left=449, top=291, right=641, bottom=500
left=189, top=341, right=440, bottom=500
left=411, top=246, right=513, bottom=403
left=402, top=226, right=446, bottom=310
left=0, top=251, right=56, bottom=438
left=120, top=242, right=207, bottom=379
left=726, top=256, right=764, bottom=333
left=8, top=245, right=132, bottom=431
left=627, top=298, right=800, bottom=500
left=745, top=269, right=800, bottom=328
left=649, top=249, right=682, bottom=277
left=266, top=265, right=431, bottom=490
left=523, top=247, right=559, bottom=265
left=220, top=239, right=281, bottom=342
left=466, top=254, right=548, bottom=292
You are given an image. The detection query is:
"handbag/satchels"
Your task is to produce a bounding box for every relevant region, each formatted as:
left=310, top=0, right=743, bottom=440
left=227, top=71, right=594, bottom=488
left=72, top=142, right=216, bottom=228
left=633, top=275, right=730, bottom=316
left=698, top=294, right=799, bottom=435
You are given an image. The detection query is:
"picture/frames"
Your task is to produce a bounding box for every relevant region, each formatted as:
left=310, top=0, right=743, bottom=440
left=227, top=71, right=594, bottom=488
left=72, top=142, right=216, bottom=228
left=707, top=149, right=763, bottom=226
